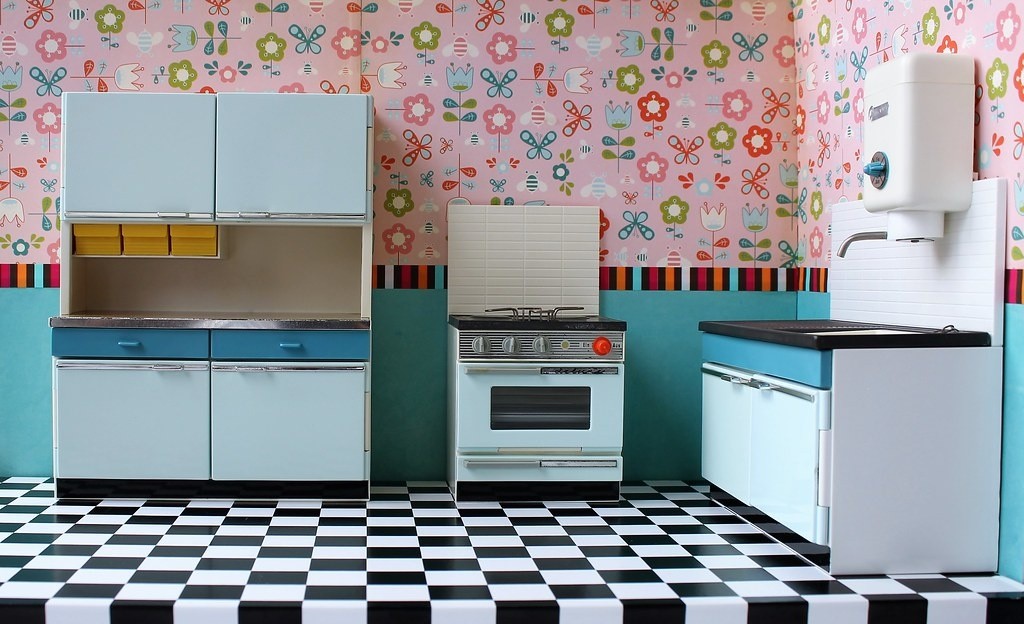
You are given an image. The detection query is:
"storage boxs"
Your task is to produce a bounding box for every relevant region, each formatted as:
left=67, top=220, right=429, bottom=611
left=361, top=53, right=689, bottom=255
left=73, top=224, right=122, bottom=255
left=170, top=224, right=217, bottom=256
left=122, top=224, right=169, bottom=256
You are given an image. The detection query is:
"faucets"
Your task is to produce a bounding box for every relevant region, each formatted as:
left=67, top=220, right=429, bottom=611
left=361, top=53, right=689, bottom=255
left=836, top=231, right=887, bottom=257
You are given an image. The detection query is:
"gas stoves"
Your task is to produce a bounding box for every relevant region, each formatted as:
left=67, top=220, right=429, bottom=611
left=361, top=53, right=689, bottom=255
left=445, top=304, right=627, bottom=360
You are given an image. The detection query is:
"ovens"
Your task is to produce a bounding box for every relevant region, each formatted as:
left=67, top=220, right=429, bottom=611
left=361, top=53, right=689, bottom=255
left=447, top=364, right=625, bottom=501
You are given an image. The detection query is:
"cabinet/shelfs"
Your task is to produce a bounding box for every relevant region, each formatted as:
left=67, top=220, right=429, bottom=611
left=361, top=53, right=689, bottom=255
left=52, top=327, right=371, bottom=360
left=701, top=334, right=1004, bottom=576
left=56, top=359, right=366, bottom=482
left=60, top=93, right=365, bottom=225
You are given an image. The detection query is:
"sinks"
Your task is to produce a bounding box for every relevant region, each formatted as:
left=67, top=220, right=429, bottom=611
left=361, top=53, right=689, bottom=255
left=774, top=326, right=932, bottom=336
